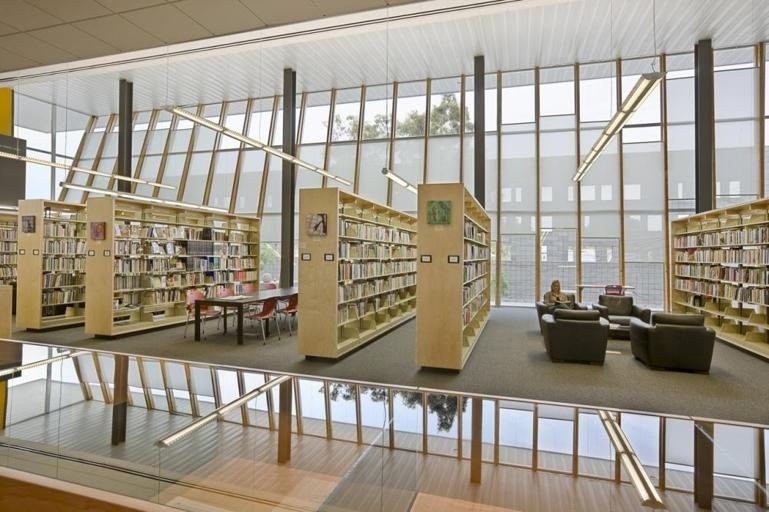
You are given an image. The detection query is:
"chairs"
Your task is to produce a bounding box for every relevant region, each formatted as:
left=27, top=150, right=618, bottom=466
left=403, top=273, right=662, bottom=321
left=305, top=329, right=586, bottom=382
left=535, top=291, right=716, bottom=375
left=247, top=283, right=298, bottom=346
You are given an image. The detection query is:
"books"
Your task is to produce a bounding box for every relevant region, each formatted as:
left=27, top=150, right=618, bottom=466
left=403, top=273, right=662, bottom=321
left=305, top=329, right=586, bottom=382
left=338, top=219, right=416, bottom=322
left=0, top=222, right=18, bottom=284
left=114, top=221, right=258, bottom=312
left=42, top=222, right=85, bottom=304
left=463, top=217, right=488, bottom=325
left=674, top=226, right=769, bottom=306
left=555, top=300, right=570, bottom=304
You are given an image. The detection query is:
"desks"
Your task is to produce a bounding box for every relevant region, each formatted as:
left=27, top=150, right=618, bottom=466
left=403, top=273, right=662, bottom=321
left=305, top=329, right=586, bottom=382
left=576, top=284, right=635, bottom=303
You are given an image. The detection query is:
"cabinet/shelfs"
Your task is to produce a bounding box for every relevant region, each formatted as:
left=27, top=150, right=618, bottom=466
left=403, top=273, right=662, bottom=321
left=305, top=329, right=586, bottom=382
left=297, top=182, right=492, bottom=374
left=0, top=340, right=769, bottom=512
left=0, top=196, right=261, bottom=340
left=667, top=197, right=768, bottom=360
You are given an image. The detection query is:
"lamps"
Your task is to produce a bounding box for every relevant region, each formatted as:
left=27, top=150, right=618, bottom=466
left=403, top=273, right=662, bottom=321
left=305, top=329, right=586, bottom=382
left=0, top=0, right=667, bottom=214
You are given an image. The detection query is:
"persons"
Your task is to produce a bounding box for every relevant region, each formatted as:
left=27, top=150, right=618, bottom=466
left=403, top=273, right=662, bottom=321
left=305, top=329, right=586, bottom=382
left=544, top=280, right=569, bottom=313
